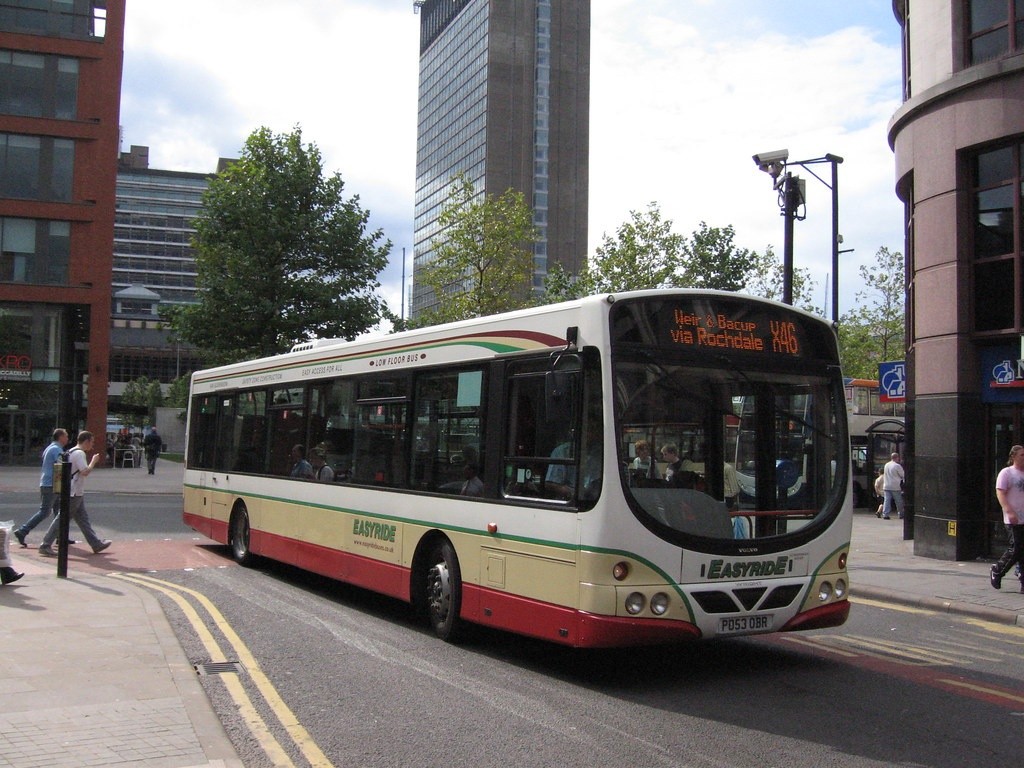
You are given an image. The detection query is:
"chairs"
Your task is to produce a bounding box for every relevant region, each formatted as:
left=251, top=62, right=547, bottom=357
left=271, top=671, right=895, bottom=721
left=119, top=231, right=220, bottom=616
left=122, top=451, right=134, bottom=468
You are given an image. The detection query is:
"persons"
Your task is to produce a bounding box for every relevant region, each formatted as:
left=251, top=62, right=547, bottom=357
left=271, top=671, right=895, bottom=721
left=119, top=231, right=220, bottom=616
left=873, top=452, right=905, bottom=519
left=632, top=440, right=683, bottom=481
left=990, top=444, right=1024, bottom=594
left=287, top=443, right=336, bottom=482
left=142, top=425, right=163, bottom=474
left=723, top=462, right=740, bottom=509
left=107, top=432, right=144, bottom=466
left=457, top=463, right=482, bottom=496
left=0, top=516, right=26, bottom=588
left=543, top=411, right=603, bottom=503
left=14, top=428, right=76, bottom=548
left=38, top=431, right=112, bottom=557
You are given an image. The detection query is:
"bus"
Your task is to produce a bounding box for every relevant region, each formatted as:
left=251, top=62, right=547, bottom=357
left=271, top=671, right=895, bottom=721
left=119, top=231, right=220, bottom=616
left=733, top=379, right=905, bottom=508
left=183, top=288, right=853, bottom=649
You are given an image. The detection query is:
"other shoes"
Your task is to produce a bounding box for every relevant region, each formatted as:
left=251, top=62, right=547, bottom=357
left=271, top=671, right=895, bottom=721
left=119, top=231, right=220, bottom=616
left=0, top=567, right=25, bottom=585
left=94, top=540, right=112, bottom=553
left=148, top=470, right=154, bottom=474
left=38, top=543, right=57, bottom=556
left=13, top=529, right=26, bottom=546
left=884, top=516, right=890, bottom=519
left=875, top=512, right=881, bottom=518
left=56, top=538, right=75, bottom=544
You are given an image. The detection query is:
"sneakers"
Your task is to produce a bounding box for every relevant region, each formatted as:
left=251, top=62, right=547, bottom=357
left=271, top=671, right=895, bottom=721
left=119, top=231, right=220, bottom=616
left=990, top=564, right=1001, bottom=589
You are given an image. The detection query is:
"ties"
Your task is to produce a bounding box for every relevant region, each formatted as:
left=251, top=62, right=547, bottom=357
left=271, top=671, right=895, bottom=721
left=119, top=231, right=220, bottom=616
left=464, top=481, right=470, bottom=495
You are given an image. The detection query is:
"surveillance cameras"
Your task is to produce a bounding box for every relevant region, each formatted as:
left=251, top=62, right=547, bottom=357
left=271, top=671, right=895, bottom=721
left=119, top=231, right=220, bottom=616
left=752, top=149, right=789, bottom=165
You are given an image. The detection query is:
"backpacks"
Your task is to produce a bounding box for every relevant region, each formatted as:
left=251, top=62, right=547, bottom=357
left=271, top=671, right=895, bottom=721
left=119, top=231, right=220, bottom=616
left=53, top=448, right=86, bottom=494
